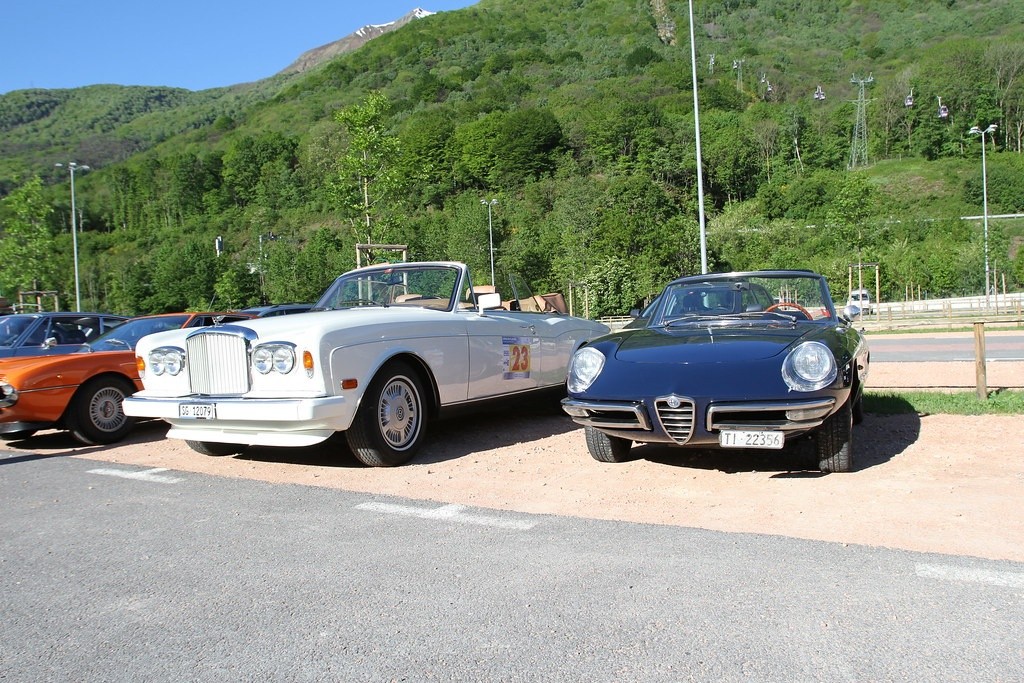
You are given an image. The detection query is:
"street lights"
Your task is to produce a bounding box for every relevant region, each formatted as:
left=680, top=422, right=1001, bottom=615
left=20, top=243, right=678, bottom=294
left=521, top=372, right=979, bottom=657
left=480, top=198, right=499, bottom=286
left=54, top=161, right=90, bottom=330
left=968, top=125, right=998, bottom=312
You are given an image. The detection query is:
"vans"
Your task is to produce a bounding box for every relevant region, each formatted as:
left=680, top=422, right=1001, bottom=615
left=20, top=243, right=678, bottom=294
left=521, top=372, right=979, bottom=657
left=847, top=290, right=872, bottom=315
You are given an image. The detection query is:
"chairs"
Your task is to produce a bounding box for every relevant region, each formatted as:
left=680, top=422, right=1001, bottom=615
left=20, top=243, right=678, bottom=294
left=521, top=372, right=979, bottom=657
left=42, top=332, right=61, bottom=344
left=465, top=286, right=496, bottom=303
left=683, top=294, right=701, bottom=311
left=395, top=294, right=422, bottom=303
left=63, top=329, right=87, bottom=344
left=746, top=304, right=763, bottom=312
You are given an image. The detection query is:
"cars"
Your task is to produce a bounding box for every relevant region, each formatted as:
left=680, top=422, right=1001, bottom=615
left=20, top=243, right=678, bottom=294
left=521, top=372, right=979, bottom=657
left=557, top=268, right=870, bottom=473
left=0, top=311, right=130, bottom=442
left=241, top=301, right=319, bottom=318
left=120, top=261, right=611, bottom=468
left=0, top=311, right=260, bottom=446
